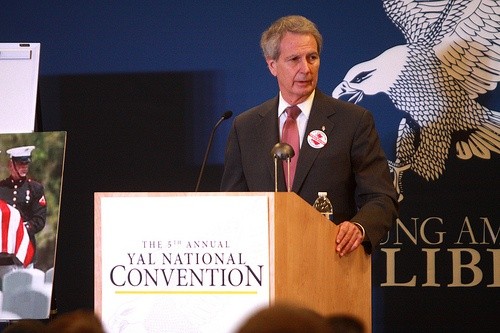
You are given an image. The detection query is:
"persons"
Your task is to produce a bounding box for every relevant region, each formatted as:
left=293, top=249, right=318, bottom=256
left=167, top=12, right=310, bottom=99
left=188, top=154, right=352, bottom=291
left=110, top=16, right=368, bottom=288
left=220, top=14, right=399, bottom=257
left=0, top=146, right=46, bottom=250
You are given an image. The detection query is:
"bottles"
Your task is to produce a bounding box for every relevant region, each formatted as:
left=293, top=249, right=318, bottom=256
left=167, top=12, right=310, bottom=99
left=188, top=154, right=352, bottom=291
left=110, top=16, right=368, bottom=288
left=312, top=192, right=333, bottom=221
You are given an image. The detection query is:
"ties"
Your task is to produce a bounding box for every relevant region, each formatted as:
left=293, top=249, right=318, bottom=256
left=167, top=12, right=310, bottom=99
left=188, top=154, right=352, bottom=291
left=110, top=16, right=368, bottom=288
left=282, top=106, right=302, bottom=192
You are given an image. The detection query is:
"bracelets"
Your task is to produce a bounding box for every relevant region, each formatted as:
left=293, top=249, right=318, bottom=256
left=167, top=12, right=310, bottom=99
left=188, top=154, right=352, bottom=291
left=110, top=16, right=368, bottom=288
left=352, top=222, right=365, bottom=242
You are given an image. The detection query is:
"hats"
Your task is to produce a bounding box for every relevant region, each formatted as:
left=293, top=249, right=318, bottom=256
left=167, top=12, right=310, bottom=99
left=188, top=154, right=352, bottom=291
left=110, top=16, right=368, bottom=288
left=6, top=146, right=35, bottom=162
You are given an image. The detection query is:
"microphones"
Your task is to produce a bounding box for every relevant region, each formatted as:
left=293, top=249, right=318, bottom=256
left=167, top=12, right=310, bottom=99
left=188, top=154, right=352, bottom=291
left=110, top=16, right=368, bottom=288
left=195, top=110, right=233, bottom=192
